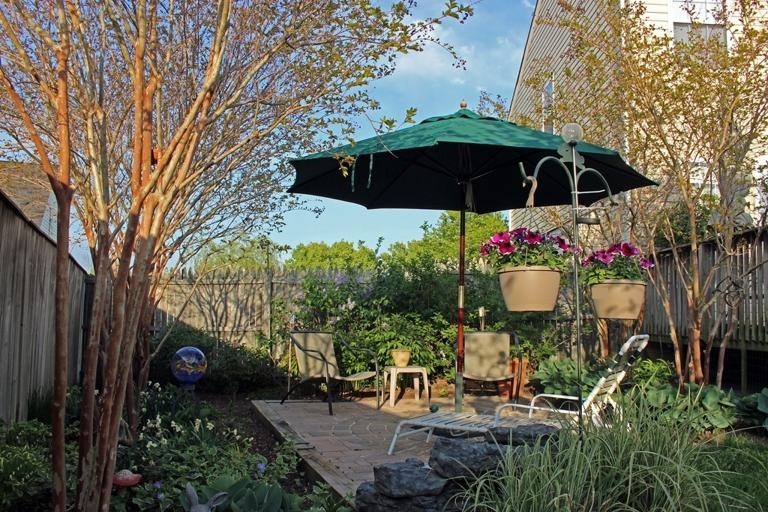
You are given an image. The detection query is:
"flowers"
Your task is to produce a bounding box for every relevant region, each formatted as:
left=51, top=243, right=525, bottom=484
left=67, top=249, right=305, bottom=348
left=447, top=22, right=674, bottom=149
left=482, top=231, right=655, bottom=287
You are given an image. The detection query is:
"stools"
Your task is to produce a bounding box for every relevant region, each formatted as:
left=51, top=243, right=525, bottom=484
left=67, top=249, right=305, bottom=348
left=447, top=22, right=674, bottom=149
left=382, top=365, right=430, bottom=411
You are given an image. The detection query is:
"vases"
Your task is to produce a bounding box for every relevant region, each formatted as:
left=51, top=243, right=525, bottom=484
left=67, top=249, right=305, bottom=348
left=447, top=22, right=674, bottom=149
left=496, top=265, right=647, bottom=321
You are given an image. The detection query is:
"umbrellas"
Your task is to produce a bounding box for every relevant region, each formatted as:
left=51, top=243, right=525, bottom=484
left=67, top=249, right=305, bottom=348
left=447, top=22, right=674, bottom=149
left=284, top=100, right=660, bottom=414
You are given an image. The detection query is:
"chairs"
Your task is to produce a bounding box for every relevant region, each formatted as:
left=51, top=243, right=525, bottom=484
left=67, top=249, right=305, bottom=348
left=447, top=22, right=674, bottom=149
left=387, top=328, right=648, bottom=462
left=280, top=333, right=380, bottom=415
